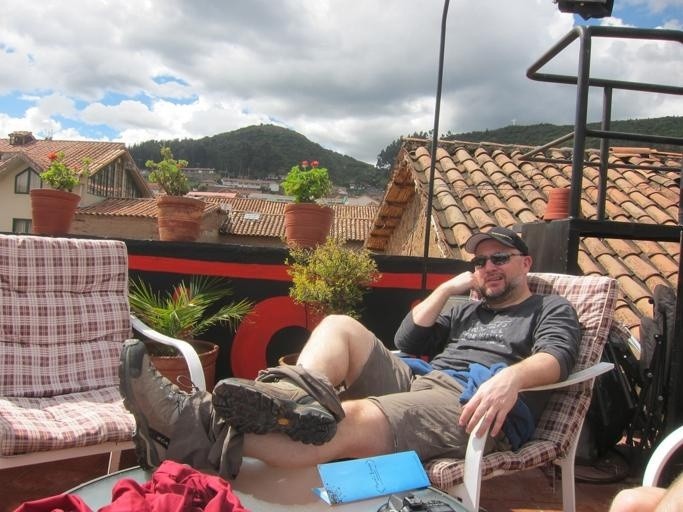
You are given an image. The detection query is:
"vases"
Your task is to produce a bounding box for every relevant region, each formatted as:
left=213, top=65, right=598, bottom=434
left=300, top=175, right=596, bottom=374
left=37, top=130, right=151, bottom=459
left=30, top=188, right=82, bottom=237
left=284, top=201, right=336, bottom=252
left=543, top=188, right=572, bottom=220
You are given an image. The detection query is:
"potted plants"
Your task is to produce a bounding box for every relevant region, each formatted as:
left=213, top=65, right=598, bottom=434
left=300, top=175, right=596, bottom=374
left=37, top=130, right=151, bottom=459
left=279, top=237, right=384, bottom=368
left=127, top=273, right=260, bottom=395
left=145, top=144, right=205, bottom=243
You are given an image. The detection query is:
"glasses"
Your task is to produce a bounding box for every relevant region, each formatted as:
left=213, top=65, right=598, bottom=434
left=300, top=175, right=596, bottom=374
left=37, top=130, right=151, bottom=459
left=471, top=253, right=527, bottom=267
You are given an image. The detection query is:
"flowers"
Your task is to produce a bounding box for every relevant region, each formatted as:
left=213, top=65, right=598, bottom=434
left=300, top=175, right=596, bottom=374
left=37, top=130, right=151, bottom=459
left=279, top=159, right=333, bottom=204
left=40, top=151, right=94, bottom=192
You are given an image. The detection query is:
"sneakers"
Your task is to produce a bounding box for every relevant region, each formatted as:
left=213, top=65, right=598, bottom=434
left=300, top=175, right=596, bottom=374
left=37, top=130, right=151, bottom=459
left=212, top=374, right=336, bottom=445
left=118, top=339, right=199, bottom=473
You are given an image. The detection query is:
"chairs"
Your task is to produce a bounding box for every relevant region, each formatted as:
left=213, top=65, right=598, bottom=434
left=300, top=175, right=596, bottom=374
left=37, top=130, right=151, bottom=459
left=415, top=271, right=619, bottom=512
left=0, top=233, right=139, bottom=475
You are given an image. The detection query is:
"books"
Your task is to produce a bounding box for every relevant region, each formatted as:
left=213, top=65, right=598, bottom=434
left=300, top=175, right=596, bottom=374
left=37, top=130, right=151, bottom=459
left=308, top=451, right=430, bottom=505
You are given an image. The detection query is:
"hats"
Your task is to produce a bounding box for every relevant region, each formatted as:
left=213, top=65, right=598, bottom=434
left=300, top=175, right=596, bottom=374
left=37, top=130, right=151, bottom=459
left=465, top=227, right=528, bottom=256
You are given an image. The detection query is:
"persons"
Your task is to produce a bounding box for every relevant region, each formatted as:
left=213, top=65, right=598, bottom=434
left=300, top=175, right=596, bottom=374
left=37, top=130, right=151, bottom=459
left=607, top=470, right=683, bottom=511
left=117, top=226, right=581, bottom=482
